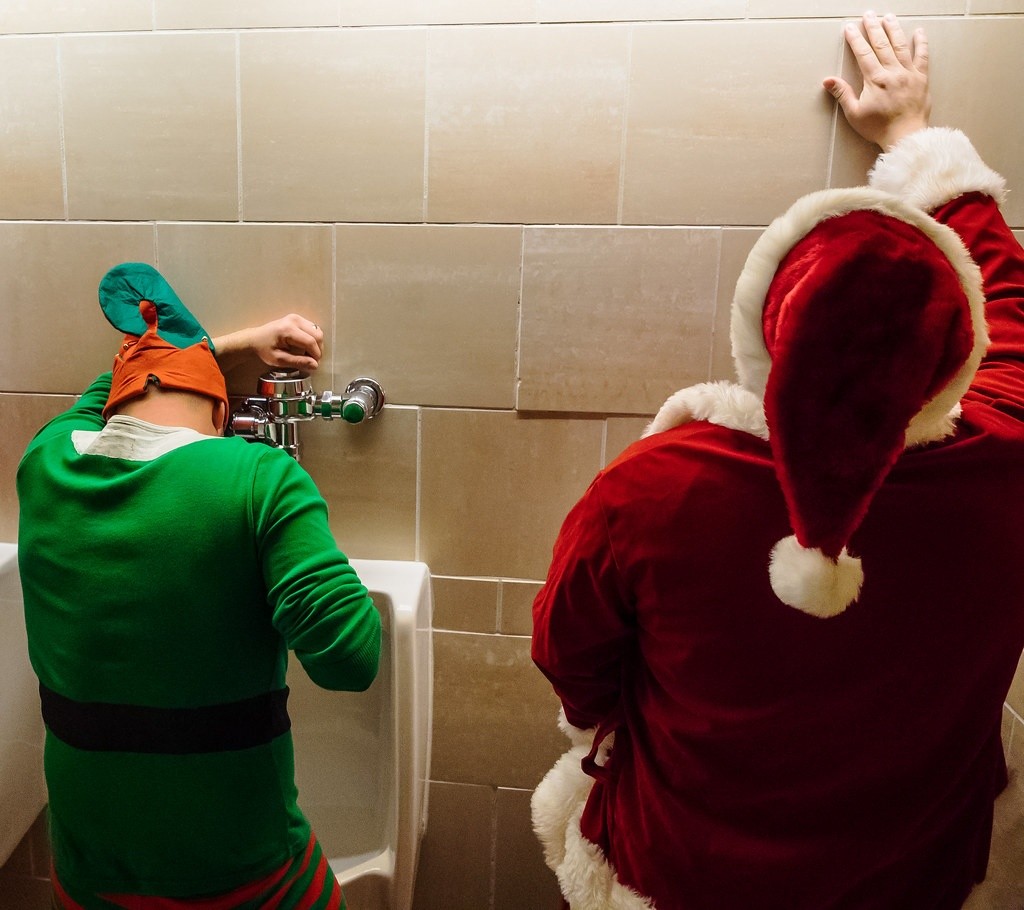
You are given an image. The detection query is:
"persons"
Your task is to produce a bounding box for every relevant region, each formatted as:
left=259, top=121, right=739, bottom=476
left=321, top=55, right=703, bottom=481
left=14, top=261, right=387, bottom=910
left=530, top=9, right=1024, bottom=910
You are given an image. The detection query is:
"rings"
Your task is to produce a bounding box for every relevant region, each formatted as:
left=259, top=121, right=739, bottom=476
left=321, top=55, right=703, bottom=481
left=313, top=325, right=317, bottom=329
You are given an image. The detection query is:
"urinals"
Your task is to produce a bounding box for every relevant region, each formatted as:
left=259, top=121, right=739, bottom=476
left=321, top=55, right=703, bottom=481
left=0, top=545, right=51, bottom=863
left=287, top=559, right=436, bottom=909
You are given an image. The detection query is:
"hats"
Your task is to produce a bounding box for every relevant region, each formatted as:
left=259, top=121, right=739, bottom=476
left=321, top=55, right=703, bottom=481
left=728, top=186, right=989, bottom=618
left=98, top=262, right=229, bottom=434
